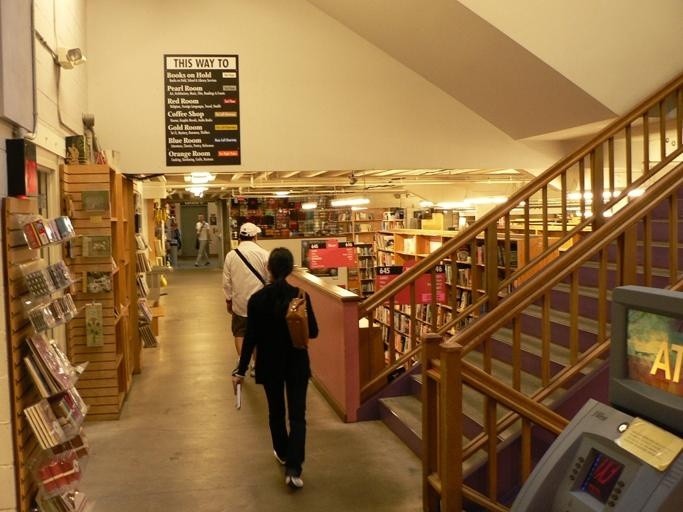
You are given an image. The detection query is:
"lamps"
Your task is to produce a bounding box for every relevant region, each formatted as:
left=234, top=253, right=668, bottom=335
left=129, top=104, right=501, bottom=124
left=52, top=37, right=86, bottom=72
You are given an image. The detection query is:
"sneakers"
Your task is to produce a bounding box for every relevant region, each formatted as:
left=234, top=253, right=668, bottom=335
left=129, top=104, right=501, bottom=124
left=250, top=366, right=256, bottom=377
left=285, top=475, right=304, bottom=488
left=231, top=364, right=240, bottom=375
left=273, top=450, right=288, bottom=465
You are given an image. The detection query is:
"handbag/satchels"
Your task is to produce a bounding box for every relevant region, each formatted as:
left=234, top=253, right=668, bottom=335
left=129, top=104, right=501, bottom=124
left=285, top=286, right=310, bottom=347
left=195, top=238, right=200, bottom=249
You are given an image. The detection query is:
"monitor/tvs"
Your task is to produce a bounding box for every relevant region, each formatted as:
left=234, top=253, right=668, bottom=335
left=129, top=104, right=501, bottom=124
left=609, top=285, right=683, bottom=432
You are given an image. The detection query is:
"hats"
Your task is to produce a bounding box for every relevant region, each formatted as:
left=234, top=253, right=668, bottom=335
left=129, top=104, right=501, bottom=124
left=239, top=222, right=263, bottom=237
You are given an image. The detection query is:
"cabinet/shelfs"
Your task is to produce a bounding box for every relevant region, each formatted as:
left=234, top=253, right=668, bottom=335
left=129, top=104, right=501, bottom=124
left=220, top=189, right=606, bottom=384
left=2, top=194, right=94, bottom=511
left=130, top=174, right=172, bottom=349
left=59, top=163, right=141, bottom=421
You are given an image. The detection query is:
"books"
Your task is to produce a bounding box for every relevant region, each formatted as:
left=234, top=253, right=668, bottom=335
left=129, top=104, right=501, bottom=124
left=135, top=235, right=157, bottom=347
left=383, top=221, right=403, bottom=229
left=233, top=383, right=242, bottom=410
left=24, top=334, right=88, bottom=512
left=64, top=134, right=87, bottom=165
left=357, top=235, right=517, bottom=373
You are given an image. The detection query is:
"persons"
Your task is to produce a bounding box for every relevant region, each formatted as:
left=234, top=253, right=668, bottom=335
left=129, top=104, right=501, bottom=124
left=222, top=223, right=271, bottom=377
left=194, top=213, right=211, bottom=267
left=232, top=247, right=318, bottom=490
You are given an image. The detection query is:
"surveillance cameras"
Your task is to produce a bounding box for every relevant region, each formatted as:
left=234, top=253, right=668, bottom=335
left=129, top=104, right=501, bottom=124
left=350, top=178, right=355, bottom=184
